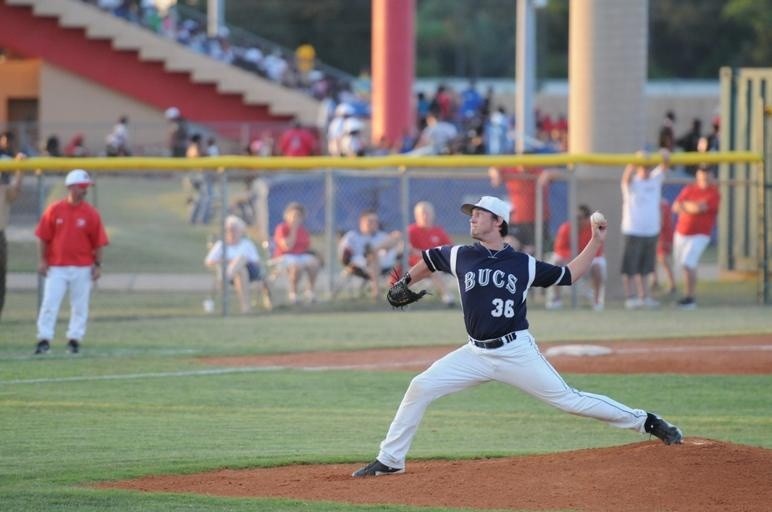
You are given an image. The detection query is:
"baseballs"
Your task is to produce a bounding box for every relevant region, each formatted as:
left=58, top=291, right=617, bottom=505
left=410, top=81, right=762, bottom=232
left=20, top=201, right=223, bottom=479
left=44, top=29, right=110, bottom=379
left=589, top=212, right=605, bottom=227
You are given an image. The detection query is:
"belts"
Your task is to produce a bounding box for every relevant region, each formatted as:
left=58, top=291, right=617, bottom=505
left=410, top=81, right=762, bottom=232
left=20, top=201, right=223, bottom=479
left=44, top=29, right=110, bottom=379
left=469, top=332, right=517, bottom=350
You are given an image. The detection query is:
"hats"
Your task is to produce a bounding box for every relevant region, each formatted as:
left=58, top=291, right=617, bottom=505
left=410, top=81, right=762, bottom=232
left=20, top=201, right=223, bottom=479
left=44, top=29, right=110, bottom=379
left=460, top=196, right=510, bottom=226
left=64, top=169, right=95, bottom=188
left=345, top=117, right=363, bottom=134
left=333, top=102, right=356, bottom=118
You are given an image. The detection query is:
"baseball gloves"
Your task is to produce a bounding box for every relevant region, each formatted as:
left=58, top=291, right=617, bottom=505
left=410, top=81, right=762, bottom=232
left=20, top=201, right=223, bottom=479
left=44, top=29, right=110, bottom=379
left=386, top=267, right=432, bottom=310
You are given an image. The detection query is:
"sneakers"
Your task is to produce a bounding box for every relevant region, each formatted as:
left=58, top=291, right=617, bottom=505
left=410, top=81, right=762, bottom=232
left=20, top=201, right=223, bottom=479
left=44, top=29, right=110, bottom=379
left=352, top=459, right=406, bottom=478
left=35, top=339, right=51, bottom=355
left=541, top=294, right=699, bottom=310
left=64, top=340, right=80, bottom=355
left=643, top=412, right=684, bottom=446
left=240, top=291, right=458, bottom=312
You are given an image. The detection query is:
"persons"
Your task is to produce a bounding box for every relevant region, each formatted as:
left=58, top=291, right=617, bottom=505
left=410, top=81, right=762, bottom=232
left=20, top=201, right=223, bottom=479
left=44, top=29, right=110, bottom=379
left=318, top=70, right=571, bottom=156
left=338, top=211, right=404, bottom=297
left=273, top=202, right=323, bottom=303
left=1, top=116, right=131, bottom=182
left=34, top=169, right=110, bottom=354
left=618, top=148, right=720, bottom=309
left=207, top=215, right=262, bottom=314
left=490, top=162, right=553, bottom=254
left=100, top=0, right=333, bottom=99
left=166, top=107, right=317, bottom=225
left=1, top=152, right=28, bottom=313
left=544, top=205, right=608, bottom=312
left=660, top=111, right=721, bottom=180
left=408, top=201, right=454, bottom=307
left=352, top=196, right=682, bottom=479
